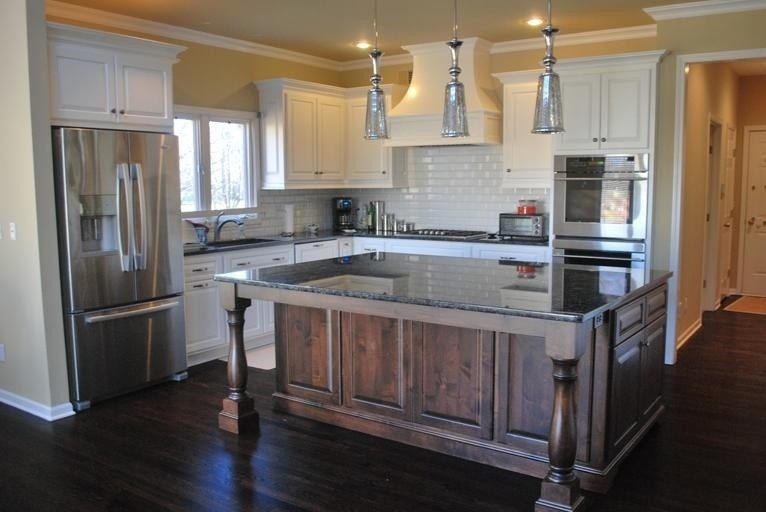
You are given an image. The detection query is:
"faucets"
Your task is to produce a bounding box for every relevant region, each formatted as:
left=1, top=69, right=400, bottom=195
left=214, top=211, right=244, bottom=241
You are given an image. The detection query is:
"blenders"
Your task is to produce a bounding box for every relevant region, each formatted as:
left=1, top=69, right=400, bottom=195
left=331, top=197, right=355, bottom=233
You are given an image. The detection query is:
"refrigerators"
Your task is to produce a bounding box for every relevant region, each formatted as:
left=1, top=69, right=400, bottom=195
left=50, top=127, right=189, bottom=411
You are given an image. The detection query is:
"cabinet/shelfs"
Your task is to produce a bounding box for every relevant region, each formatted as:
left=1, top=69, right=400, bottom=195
left=280, top=85, right=346, bottom=188
left=45, top=34, right=174, bottom=131
left=346, top=90, right=389, bottom=187
left=355, top=226, right=550, bottom=262
left=182, top=243, right=292, bottom=367
left=546, top=52, right=670, bottom=153
left=492, top=69, right=560, bottom=188
left=294, top=230, right=353, bottom=264
left=212, top=251, right=674, bottom=512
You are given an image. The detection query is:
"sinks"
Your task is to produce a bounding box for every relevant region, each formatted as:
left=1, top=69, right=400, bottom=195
left=208, top=238, right=279, bottom=247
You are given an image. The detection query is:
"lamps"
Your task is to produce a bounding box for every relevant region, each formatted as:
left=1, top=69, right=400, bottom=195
left=363, top=0, right=390, bottom=141
left=533, top=0, right=562, bottom=133
left=441, top=0, right=475, bottom=139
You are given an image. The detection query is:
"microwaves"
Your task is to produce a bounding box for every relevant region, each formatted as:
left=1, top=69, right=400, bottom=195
left=497, top=214, right=549, bottom=238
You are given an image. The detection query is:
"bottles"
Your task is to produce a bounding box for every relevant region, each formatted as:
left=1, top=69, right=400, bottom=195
left=368, top=201, right=375, bottom=234
left=522, top=265, right=536, bottom=280
left=384, top=215, right=393, bottom=236
left=518, top=201, right=527, bottom=214
left=527, top=200, right=536, bottom=214
left=361, top=205, right=368, bottom=234
left=372, top=230, right=373, bottom=232
left=393, top=220, right=402, bottom=232
left=376, top=202, right=384, bottom=235
left=516, top=264, right=524, bottom=279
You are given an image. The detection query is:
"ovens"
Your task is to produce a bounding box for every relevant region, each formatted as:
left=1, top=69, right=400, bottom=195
left=551, top=154, right=647, bottom=267
